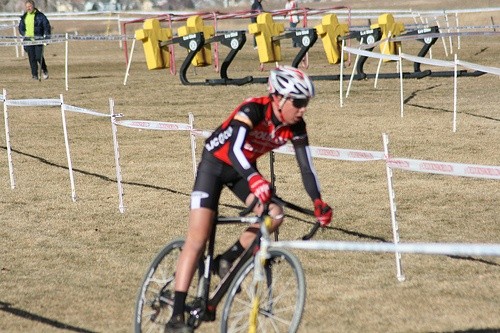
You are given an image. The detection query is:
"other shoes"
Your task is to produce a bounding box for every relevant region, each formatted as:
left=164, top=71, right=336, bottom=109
left=165, top=314, right=194, bottom=333
left=213, top=253, right=241, bottom=294
left=43, top=71, right=49, bottom=80
left=32, top=75, right=38, bottom=80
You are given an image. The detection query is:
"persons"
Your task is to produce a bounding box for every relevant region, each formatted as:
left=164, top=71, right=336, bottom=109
left=251, top=0, right=265, bottom=50
left=19, top=0, right=51, bottom=80
left=284, top=0, right=300, bottom=47
left=161, top=64, right=333, bottom=333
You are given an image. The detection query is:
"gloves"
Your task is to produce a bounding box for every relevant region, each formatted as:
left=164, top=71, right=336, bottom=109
left=248, top=174, right=275, bottom=204
left=312, top=198, right=332, bottom=227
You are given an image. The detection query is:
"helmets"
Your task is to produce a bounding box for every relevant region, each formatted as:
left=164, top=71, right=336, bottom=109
left=268, top=64, right=315, bottom=99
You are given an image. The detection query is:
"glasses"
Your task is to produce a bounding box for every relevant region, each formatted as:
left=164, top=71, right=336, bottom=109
left=292, top=97, right=310, bottom=108
